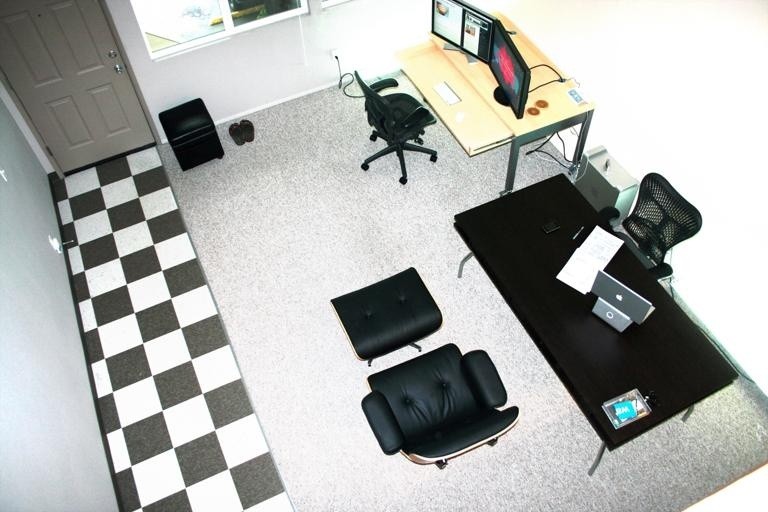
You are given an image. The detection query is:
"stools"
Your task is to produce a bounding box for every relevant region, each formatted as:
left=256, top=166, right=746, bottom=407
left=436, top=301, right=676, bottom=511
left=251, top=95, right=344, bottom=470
left=158, top=98, right=224, bottom=170
left=329, top=266, right=443, bottom=367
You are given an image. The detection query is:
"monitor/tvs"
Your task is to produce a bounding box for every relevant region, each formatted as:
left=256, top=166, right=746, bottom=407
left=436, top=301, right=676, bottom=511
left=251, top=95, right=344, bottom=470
left=431, top=0, right=496, bottom=65
left=488, top=19, right=532, bottom=120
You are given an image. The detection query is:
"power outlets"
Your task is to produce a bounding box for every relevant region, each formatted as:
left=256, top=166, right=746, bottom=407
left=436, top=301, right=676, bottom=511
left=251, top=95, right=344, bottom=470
left=331, top=50, right=341, bottom=60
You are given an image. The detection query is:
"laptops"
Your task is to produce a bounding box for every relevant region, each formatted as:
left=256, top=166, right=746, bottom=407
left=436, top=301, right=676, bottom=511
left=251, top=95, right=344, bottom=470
left=590, top=270, right=657, bottom=326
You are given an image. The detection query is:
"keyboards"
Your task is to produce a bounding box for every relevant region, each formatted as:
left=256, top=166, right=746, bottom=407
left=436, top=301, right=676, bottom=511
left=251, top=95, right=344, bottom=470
left=434, top=81, right=462, bottom=106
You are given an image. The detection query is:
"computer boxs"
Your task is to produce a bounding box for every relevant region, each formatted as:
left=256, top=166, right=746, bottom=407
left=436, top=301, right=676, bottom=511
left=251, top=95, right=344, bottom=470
left=576, top=146, right=639, bottom=228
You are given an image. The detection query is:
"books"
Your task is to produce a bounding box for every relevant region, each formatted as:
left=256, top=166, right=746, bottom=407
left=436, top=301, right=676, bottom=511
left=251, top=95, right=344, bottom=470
left=602, top=389, right=652, bottom=430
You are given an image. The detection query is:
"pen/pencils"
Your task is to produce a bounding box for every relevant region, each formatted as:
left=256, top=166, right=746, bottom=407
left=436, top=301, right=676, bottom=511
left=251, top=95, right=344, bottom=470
left=572, top=225, right=585, bottom=240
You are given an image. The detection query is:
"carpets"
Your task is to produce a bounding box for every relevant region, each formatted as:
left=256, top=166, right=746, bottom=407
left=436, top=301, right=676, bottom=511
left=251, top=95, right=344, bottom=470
left=47, top=142, right=296, bottom=512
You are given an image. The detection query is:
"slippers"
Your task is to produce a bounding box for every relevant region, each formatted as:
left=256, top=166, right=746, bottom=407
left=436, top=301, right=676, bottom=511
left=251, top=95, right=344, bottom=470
left=229, top=123, right=244, bottom=145
left=241, top=120, right=254, bottom=142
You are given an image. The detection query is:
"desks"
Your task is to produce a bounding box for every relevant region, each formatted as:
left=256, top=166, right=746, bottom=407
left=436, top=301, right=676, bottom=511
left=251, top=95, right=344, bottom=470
left=394, top=11, right=595, bottom=197
left=453, top=173, right=739, bottom=475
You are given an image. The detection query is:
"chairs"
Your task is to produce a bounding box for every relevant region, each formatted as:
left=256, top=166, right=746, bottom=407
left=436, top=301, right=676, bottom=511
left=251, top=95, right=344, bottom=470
left=362, top=341, right=522, bottom=470
left=354, top=70, right=437, bottom=184
left=598, top=173, right=703, bottom=280
